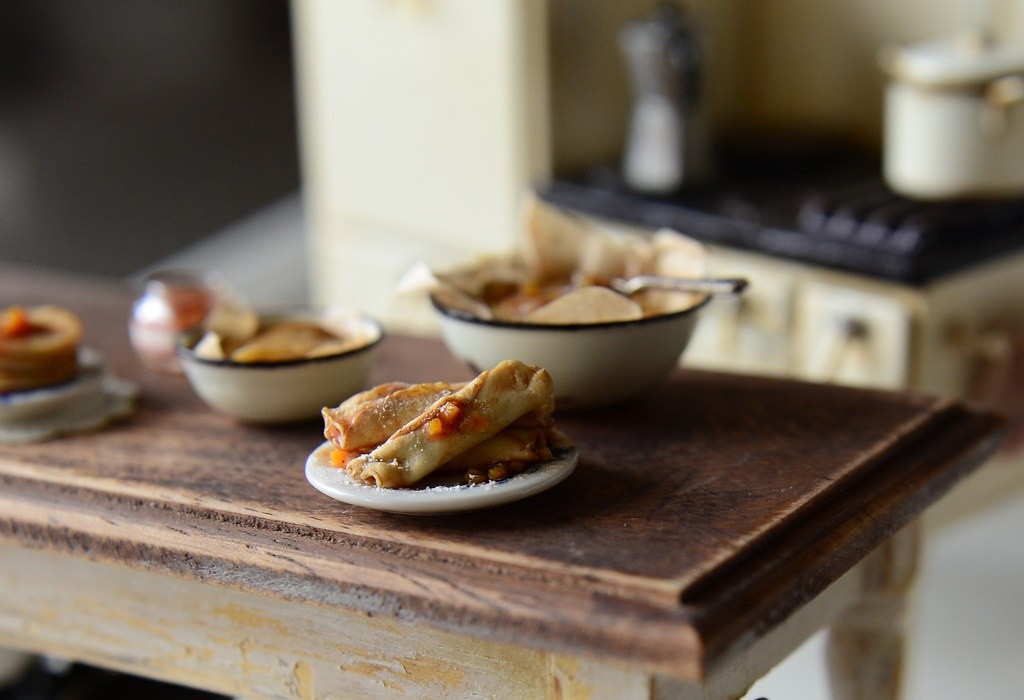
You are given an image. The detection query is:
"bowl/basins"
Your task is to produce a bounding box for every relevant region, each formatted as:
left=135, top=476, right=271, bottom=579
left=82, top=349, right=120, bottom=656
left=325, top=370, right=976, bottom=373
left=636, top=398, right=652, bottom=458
left=425, top=273, right=708, bottom=407
left=177, top=304, right=385, bottom=423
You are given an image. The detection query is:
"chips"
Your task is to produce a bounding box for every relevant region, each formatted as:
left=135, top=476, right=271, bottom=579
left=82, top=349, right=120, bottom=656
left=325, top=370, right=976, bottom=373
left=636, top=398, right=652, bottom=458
left=394, top=196, right=712, bottom=324
left=196, top=300, right=370, bottom=362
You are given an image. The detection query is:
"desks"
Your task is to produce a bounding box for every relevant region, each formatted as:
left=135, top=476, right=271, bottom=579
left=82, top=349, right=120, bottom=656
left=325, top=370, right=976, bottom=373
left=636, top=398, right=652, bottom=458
left=0, top=268, right=1007, bottom=700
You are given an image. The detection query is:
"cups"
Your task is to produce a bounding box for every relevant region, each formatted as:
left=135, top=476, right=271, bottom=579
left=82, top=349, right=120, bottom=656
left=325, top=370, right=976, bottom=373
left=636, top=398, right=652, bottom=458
left=877, top=29, right=1023, bottom=200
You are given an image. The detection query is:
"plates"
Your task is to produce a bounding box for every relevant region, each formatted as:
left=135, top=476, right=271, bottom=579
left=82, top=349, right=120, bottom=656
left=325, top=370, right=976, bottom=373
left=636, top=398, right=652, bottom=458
left=307, top=440, right=582, bottom=512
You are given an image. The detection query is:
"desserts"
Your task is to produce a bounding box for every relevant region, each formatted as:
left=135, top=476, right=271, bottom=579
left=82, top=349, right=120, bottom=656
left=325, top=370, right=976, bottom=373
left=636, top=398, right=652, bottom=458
left=0, top=304, right=83, bottom=397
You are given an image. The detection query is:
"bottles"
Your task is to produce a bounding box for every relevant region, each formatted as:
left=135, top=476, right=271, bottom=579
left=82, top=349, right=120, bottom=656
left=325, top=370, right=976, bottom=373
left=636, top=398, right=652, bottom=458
left=130, top=270, right=211, bottom=371
left=619, top=5, right=704, bottom=193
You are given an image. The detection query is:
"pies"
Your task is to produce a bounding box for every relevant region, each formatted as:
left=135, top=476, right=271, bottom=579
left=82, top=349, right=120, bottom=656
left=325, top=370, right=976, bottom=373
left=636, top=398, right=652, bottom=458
left=322, top=360, right=579, bottom=491
